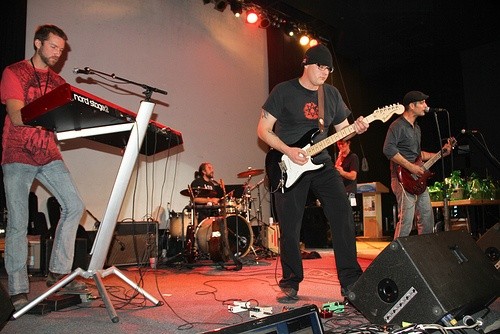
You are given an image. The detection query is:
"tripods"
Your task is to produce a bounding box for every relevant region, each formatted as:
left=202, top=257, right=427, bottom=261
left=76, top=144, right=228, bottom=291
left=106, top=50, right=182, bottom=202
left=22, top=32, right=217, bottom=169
left=167, top=206, right=193, bottom=265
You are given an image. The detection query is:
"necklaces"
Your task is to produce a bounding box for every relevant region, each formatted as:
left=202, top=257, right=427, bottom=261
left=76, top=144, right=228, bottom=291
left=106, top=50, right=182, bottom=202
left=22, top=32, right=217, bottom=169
left=30, top=56, right=50, bottom=97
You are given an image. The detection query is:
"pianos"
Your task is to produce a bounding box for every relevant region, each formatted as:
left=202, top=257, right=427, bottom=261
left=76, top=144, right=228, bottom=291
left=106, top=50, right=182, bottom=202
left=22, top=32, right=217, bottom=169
left=10, top=81, right=182, bottom=324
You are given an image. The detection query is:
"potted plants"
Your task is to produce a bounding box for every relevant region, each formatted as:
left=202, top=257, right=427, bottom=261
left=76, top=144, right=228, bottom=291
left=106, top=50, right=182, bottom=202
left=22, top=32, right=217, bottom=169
left=424, top=171, right=500, bottom=200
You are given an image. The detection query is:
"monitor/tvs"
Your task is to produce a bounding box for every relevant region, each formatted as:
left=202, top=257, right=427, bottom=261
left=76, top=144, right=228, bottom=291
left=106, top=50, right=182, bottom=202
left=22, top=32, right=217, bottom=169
left=204, top=304, right=324, bottom=334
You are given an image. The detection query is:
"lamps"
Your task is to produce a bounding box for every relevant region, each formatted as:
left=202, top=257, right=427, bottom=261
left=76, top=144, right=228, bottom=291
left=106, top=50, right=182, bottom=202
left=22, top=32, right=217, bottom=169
left=202, top=0, right=319, bottom=48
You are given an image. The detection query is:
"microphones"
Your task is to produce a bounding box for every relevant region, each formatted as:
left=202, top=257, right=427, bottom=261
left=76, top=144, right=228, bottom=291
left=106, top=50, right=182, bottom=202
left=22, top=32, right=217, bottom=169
left=242, top=239, right=246, bottom=243
left=461, top=129, right=478, bottom=134
left=118, top=241, right=126, bottom=251
left=74, top=68, right=95, bottom=74
left=251, top=179, right=264, bottom=192
left=425, top=107, right=446, bottom=112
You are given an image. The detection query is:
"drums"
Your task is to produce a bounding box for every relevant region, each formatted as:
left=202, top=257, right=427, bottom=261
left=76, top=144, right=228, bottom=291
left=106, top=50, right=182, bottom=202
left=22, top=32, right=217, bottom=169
left=170, top=209, right=198, bottom=239
left=189, top=205, right=242, bottom=220
left=194, top=214, right=255, bottom=261
left=224, top=199, right=244, bottom=215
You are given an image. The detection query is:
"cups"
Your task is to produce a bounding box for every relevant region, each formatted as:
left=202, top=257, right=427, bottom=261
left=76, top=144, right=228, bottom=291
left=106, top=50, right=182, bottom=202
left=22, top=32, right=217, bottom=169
left=150, top=258, right=157, bottom=268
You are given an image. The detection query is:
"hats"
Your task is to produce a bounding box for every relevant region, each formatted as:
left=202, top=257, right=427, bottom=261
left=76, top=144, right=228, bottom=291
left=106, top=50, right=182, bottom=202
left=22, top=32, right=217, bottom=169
left=302, top=44, right=333, bottom=67
left=403, top=91, right=429, bottom=106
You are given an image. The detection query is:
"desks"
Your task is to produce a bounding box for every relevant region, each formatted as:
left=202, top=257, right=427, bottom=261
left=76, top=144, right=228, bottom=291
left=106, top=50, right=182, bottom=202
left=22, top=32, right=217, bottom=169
left=430, top=199, right=500, bottom=234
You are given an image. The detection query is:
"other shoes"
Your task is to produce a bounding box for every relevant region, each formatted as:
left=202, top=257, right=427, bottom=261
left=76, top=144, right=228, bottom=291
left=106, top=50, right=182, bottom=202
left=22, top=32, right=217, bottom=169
left=11, top=293, right=28, bottom=310
left=276, top=286, right=298, bottom=303
left=45, top=271, right=88, bottom=290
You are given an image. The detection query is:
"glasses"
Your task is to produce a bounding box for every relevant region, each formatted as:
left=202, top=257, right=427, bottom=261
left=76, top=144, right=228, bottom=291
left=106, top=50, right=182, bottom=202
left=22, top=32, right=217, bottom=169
left=315, top=63, right=334, bottom=73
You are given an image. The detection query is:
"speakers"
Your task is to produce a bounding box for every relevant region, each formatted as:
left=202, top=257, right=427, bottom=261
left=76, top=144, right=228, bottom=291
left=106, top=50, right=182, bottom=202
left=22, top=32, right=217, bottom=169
left=106, top=222, right=159, bottom=267
left=0, top=283, right=14, bottom=331
left=346, top=223, right=500, bottom=331
left=45, top=238, right=87, bottom=277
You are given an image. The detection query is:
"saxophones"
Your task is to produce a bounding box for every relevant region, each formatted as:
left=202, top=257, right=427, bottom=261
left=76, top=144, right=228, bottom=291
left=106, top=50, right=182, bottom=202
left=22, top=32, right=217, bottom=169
left=335, top=149, right=343, bottom=170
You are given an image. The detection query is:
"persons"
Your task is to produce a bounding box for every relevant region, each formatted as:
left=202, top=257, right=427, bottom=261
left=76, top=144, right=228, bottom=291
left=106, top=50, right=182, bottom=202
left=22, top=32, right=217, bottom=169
left=383, top=90, right=452, bottom=242
left=0, top=24, right=87, bottom=313
left=333, top=140, right=360, bottom=208
left=190, top=162, right=233, bottom=205
left=257, top=44, right=370, bottom=304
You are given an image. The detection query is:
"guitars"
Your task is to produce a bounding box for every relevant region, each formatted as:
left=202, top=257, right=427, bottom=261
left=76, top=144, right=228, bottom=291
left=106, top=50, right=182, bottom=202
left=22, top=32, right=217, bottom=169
left=265, top=102, right=405, bottom=194
left=396, top=136, right=456, bottom=196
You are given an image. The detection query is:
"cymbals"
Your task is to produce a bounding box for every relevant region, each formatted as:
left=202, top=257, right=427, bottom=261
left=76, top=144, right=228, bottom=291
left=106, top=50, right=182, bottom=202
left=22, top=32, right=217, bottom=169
left=179, top=189, right=218, bottom=198
left=236, top=168, right=264, bottom=177
